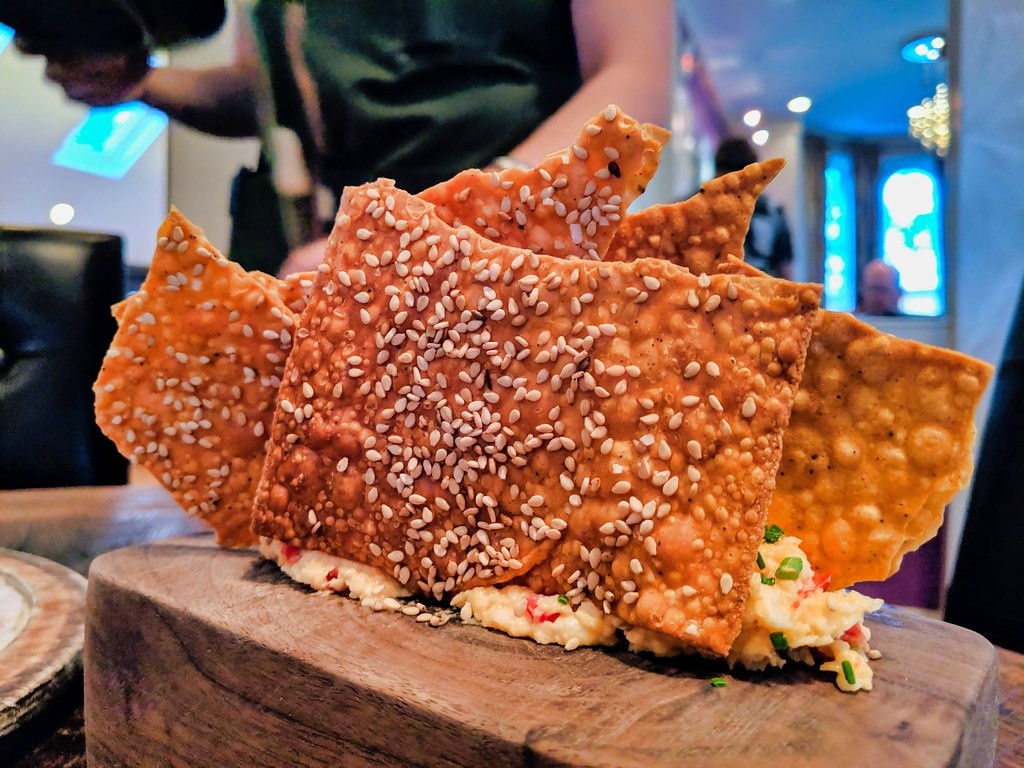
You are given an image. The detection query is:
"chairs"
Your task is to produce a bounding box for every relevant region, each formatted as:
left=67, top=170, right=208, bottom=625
left=0, top=226, right=131, bottom=490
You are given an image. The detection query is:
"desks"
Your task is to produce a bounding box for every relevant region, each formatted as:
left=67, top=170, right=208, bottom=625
left=0, top=487, right=1024, bottom=768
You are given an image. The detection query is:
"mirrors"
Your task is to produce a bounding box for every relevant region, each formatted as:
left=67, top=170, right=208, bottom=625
left=626, top=0, right=961, bottom=329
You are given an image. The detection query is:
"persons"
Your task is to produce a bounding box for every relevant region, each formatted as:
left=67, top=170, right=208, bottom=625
left=853, top=259, right=911, bottom=315
left=0, top=247, right=92, bottom=489
left=1, top=0, right=677, bottom=281
left=688, top=135, right=792, bottom=282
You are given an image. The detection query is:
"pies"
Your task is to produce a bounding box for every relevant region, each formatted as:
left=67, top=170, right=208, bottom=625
left=90, top=101, right=999, bottom=660
